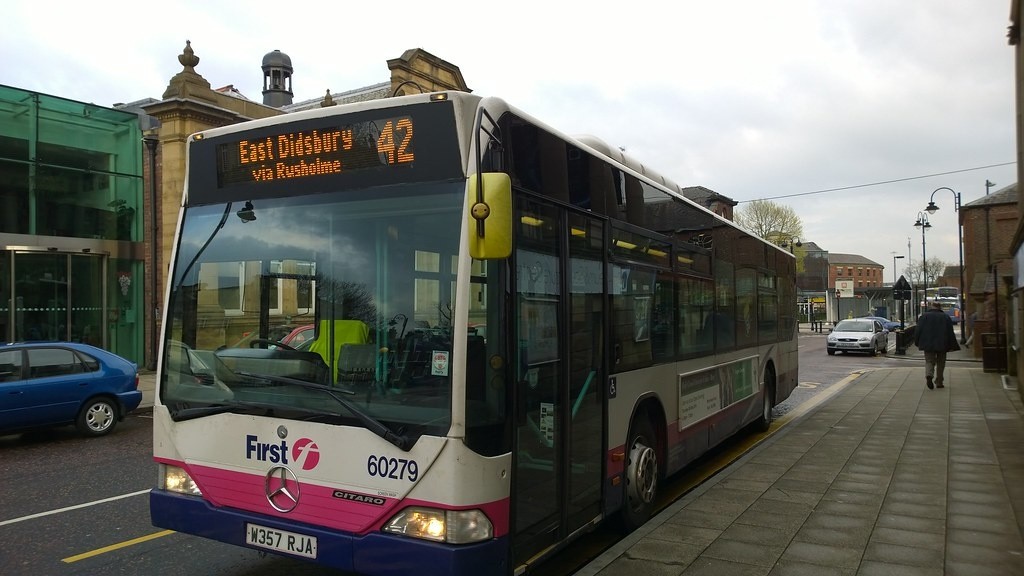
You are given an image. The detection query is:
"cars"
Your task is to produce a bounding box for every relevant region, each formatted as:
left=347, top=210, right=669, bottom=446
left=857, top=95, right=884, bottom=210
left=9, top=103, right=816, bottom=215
left=468, top=321, right=489, bottom=344
left=0, top=341, right=142, bottom=439
left=825, top=319, right=889, bottom=356
left=232, top=321, right=317, bottom=353
left=861, top=316, right=905, bottom=333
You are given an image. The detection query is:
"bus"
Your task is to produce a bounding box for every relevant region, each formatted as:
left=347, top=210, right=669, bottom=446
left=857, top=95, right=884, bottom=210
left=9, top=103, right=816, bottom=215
left=151, top=90, right=801, bottom=576
left=920, top=286, right=961, bottom=326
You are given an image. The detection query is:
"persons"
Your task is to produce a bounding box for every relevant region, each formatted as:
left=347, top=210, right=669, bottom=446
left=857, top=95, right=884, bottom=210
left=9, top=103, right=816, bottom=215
left=964, top=312, right=978, bottom=349
left=914, top=303, right=961, bottom=391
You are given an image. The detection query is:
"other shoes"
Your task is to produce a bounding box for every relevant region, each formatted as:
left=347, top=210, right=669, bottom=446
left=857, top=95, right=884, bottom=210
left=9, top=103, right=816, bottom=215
left=934, top=378, right=945, bottom=389
left=926, top=376, right=935, bottom=390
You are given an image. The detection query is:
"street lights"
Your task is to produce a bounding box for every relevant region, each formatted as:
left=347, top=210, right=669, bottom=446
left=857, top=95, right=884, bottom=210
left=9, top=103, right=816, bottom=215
left=913, top=210, right=931, bottom=312
left=924, top=187, right=967, bottom=345
left=781, top=236, right=803, bottom=255
left=892, top=255, right=905, bottom=320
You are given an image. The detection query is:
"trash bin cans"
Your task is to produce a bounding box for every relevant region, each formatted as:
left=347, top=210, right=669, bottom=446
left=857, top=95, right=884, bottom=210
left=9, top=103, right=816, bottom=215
left=979, top=331, right=1008, bottom=373
